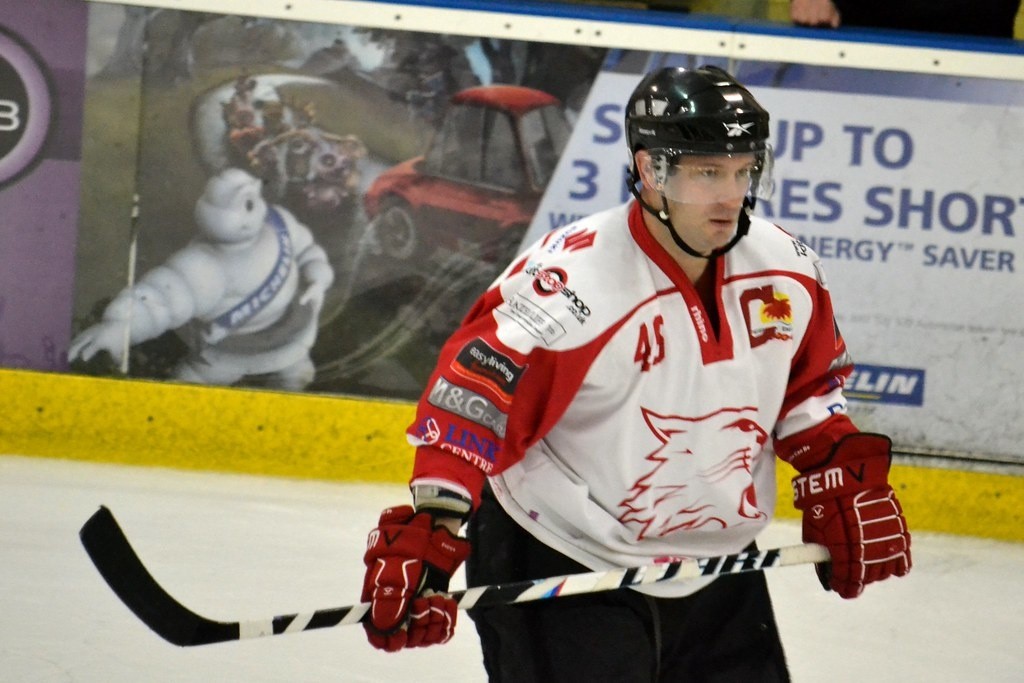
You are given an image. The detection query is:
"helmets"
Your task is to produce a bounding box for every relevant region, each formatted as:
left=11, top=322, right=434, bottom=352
left=626, top=65, right=769, bottom=182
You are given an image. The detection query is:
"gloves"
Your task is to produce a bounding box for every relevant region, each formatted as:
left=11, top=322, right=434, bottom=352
left=362, top=502, right=471, bottom=650
left=789, top=426, right=912, bottom=598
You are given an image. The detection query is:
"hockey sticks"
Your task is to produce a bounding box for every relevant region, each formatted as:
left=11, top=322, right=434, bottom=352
left=76, top=502, right=831, bottom=649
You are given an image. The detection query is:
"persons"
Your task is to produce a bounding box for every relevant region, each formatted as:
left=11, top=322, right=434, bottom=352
left=359, top=64, right=912, bottom=683
